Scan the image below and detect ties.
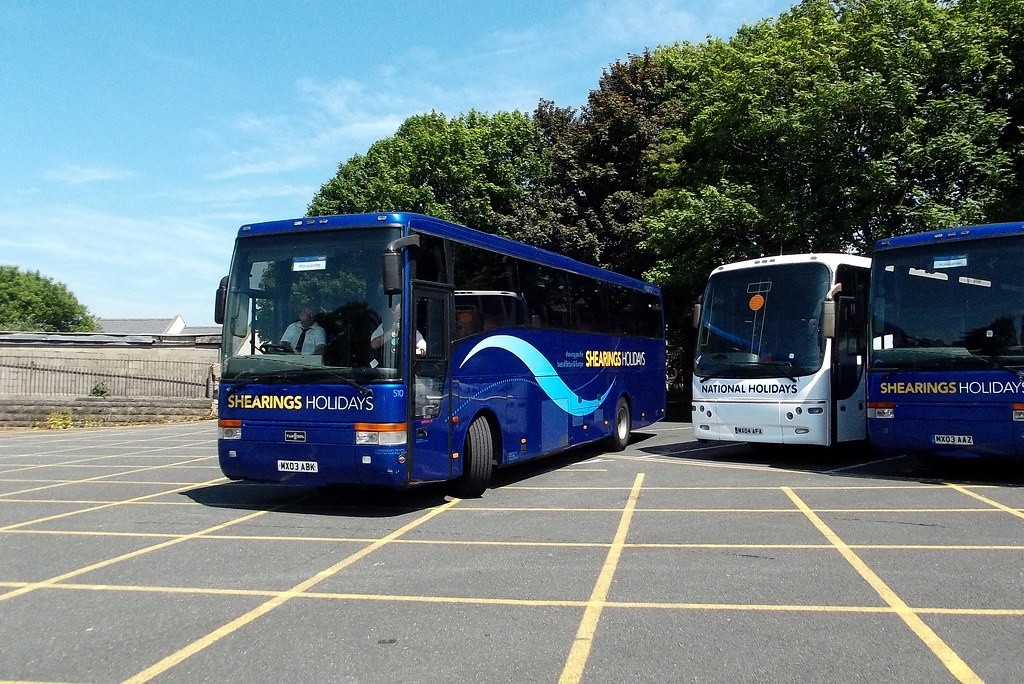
[295,328,311,353]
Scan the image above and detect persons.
[277,304,326,358]
[369,302,427,366]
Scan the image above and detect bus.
[213,211,667,505]
[863,221,1023,484]
[692,252,993,451]
